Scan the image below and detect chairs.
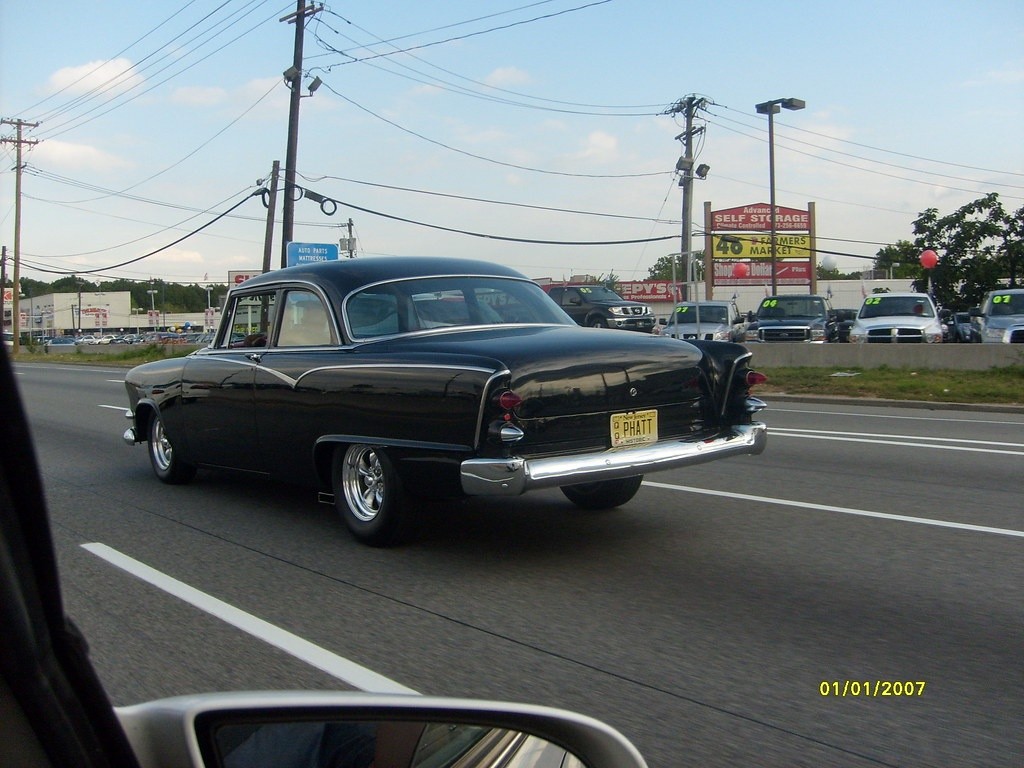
[810,305,822,315]
[770,308,786,316]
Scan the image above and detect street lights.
[205,287,212,332]
[147,290,158,333]
[755,98,805,296]
[132,308,143,335]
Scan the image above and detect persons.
[282,307,327,345]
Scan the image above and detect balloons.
[167,322,190,333]
[918,250,939,268]
[734,263,747,278]
[174,323,179,329]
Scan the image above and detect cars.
[755,294,840,342]
[969,289,1024,343]
[849,292,943,343]
[661,299,741,342]
[123,258,767,544]
[739,313,756,326]
[412,296,504,326]
[34,332,246,345]
[941,311,972,343]
[835,307,859,341]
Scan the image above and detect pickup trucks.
[491,286,660,335]
